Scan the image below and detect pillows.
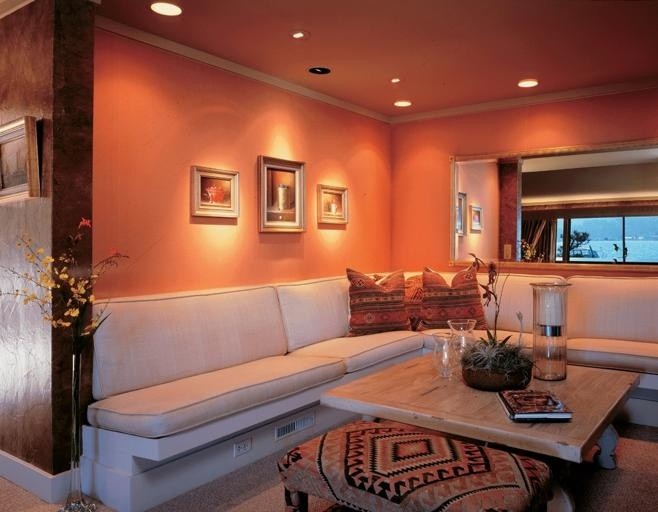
[347,267,488,337]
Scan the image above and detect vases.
[463,367,532,392]
[58,354,96,512]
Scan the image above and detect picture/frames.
[0,116,41,202]
[257,155,306,232]
[317,184,348,225]
[457,192,468,237]
[191,166,239,218]
[469,205,483,233]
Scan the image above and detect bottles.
[530,282,571,381]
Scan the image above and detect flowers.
[0,216,130,355]
[463,253,543,382]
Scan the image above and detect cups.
[432,318,475,379]
[277,187,290,210]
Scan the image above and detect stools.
[277,420,554,512]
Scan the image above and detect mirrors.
[448,138,658,273]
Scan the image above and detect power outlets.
[234,438,252,458]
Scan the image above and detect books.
[498,389,574,421]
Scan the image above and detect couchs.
[80,272,422,512]
[423,273,658,428]
